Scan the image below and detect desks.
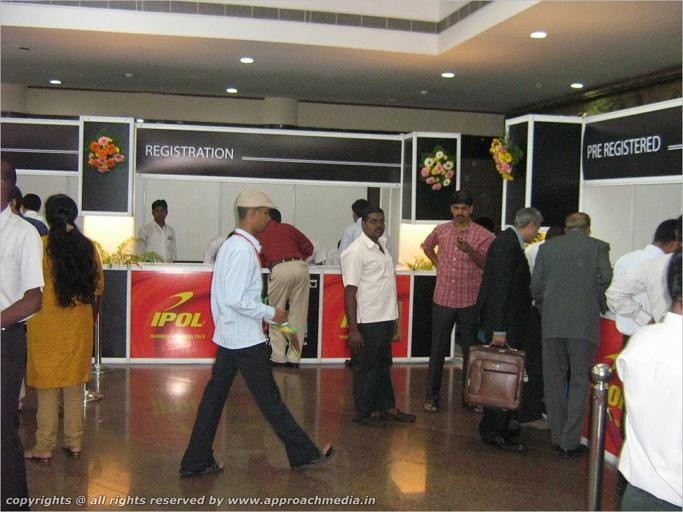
[91,262,455,362]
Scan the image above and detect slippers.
[23,447,52,467]
[61,439,81,461]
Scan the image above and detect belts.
[268,256,306,271]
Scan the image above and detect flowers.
[418,146,456,192]
[489,139,524,183]
[88,130,123,174]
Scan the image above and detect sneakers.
[356,414,389,428]
[379,406,417,422]
[518,416,550,431]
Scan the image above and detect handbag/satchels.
[464,341,529,412]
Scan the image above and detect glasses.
[364,218,386,226]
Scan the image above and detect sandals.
[179,460,225,476]
[290,444,336,472]
[423,394,439,413]
[463,403,483,414]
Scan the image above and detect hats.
[236,188,278,209]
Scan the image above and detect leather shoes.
[562,445,581,465]
[268,359,288,367]
[344,359,352,368]
[285,362,299,368]
[481,428,526,452]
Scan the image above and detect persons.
[475,208,549,450]
[8,185,48,237]
[21,193,51,232]
[422,189,496,414]
[22,193,105,467]
[604,219,683,510]
[133,188,416,477]
[531,212,614,459]
[525,226,565,276]
[605,214,682,326]
[1,155,46,511]
[614,246,683,511]
